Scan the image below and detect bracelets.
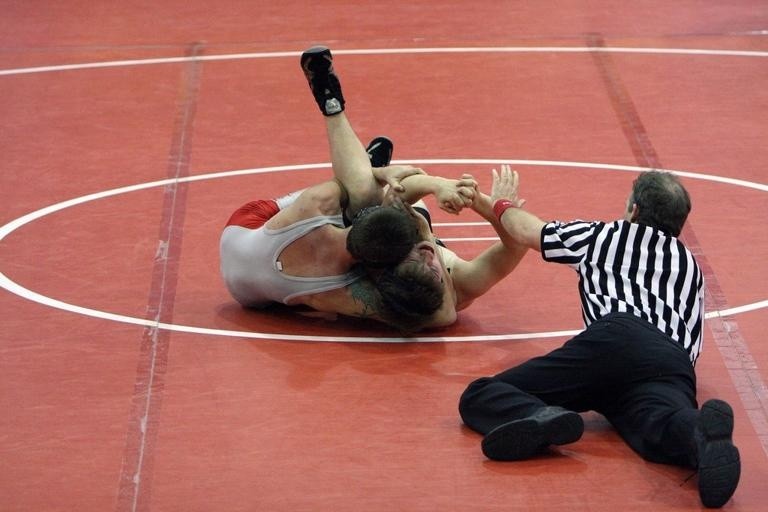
[492,198,521,225]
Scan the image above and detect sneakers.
[301,47,346,117]
[366,137,392,169]
[693,400,740,509]
[480,406,583,461]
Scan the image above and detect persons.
[296,43,526,325]
[457,163,742,511]
[217,135,479,329]
